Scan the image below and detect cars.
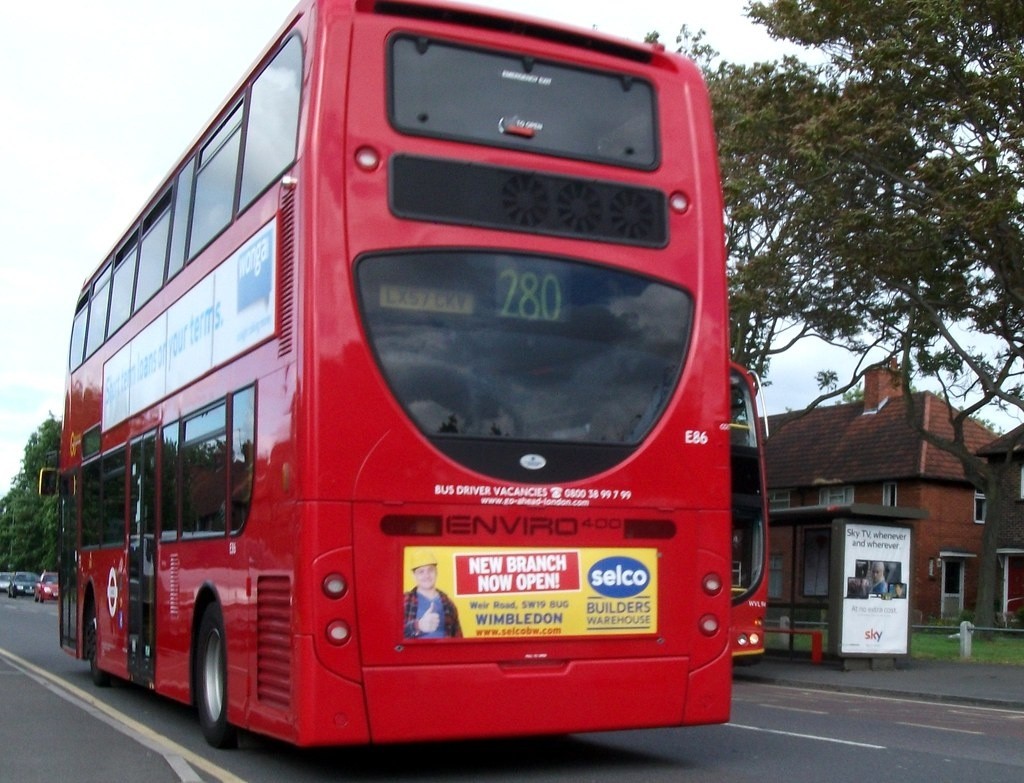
[0,572,12,591]
[35,571,60,603]
[8,571,42,598]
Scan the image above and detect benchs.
[765,626,824,665]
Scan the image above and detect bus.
[724,360,770,667]
[38,0,733,749]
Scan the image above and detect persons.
[849,578,868,598]
[894,582,906,598]
[866,562,888,595]
[402,548,464,639]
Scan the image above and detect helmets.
[409,550,437,570]
